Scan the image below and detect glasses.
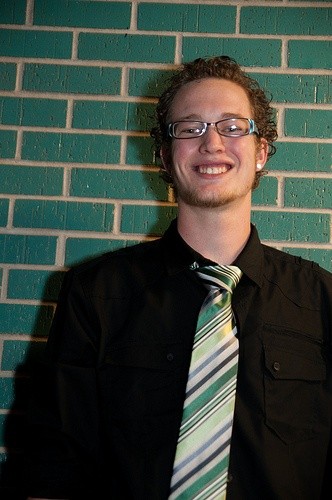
[164,117,260,139]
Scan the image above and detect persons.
[32,55,332,500]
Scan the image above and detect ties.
[165,262,242,499]
